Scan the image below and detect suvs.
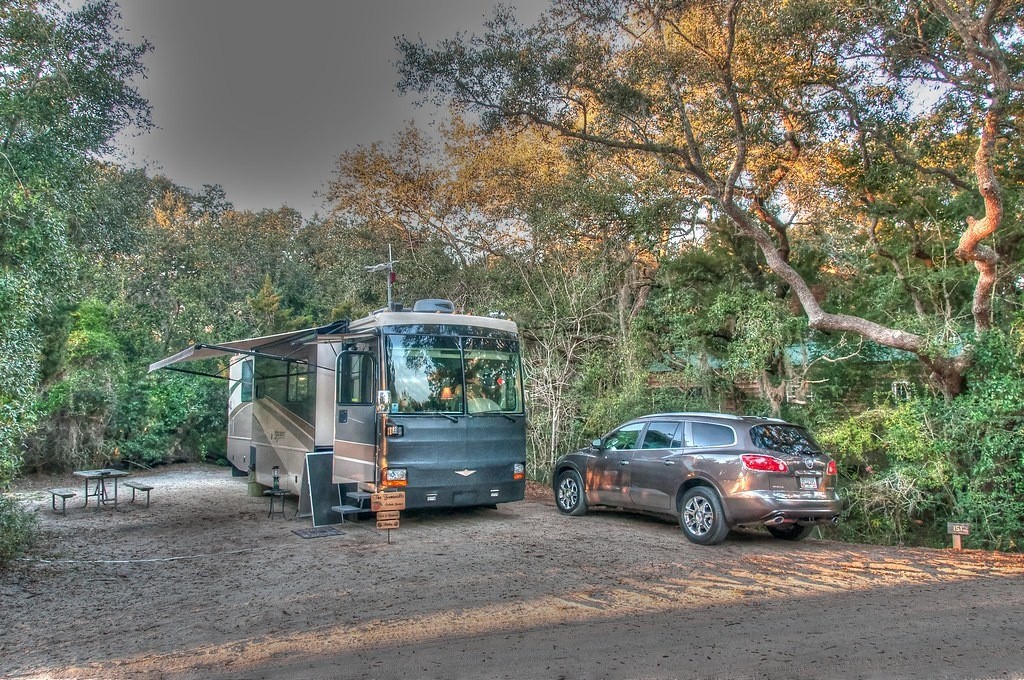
[551,412,843,547]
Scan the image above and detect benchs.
[123,481,154,508]
[48,489,76,517]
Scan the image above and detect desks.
[73,469,132,513]
[263,488,291,519]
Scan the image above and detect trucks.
[146,297,529,515]
